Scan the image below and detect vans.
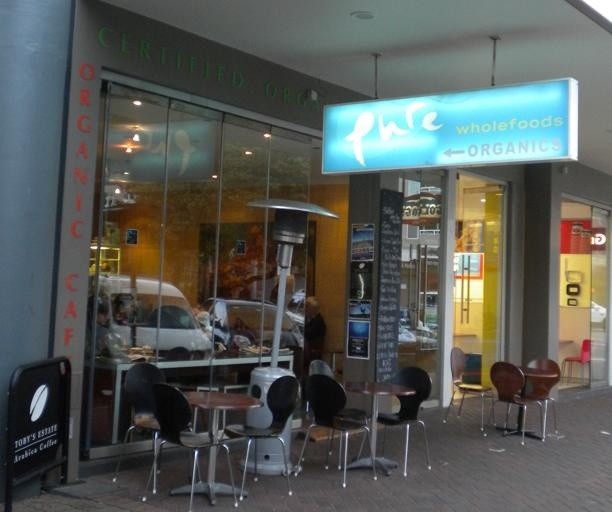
[83,274,306,395]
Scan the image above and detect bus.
[414,291,439,322]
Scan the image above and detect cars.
[396,307,440,357]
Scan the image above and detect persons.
[86,295,112,358]
[303,295,327,369]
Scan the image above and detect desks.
[169,391,264,504]
[85,349,295,445]
[346,380,417,470]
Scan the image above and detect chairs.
[440,347,497,430]
[111,363,202,485]
[294,374,377,488]
[561,338,592,385]
[308,358,371,469]
[356,365,432,476]
[223,375,302,500]
[142,384,239,510]
[485,360,562,445]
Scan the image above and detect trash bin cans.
[239,367,302,476]
